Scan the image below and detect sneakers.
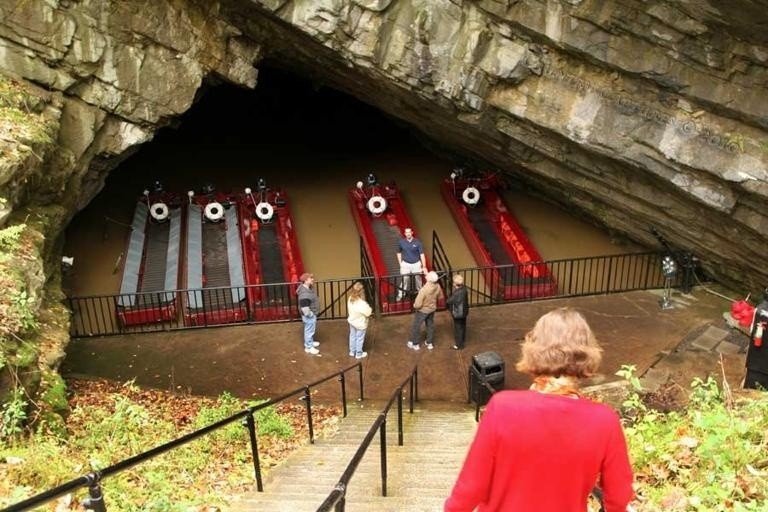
[304,341,320,354]
[349,351,367,360]
[424,340,434,350]
[407,341,420,351]
[395,296,402,302]
[453,345,458,350]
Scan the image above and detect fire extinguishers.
[754,321,767,347]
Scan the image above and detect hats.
[299,273,314,281]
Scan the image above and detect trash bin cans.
[468,351,505,406]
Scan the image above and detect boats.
[112,180,183,328]
[236,180,307,324]
[439,168,560,300]
[183,184,249,329]
[347,173,449,316]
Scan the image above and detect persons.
[297,272,322,356]
[395,227,428,302]
[412,271,444,346]
[446,274,469,350]
[347,282,373,360]
[443,306,635,512]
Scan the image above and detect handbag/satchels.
[452,304,464,319]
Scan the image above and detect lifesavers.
[203,202,225,219]
[463,188,478,203]
[367,196,387,213]
[151,203,168,220]
[256,203,273,221]
